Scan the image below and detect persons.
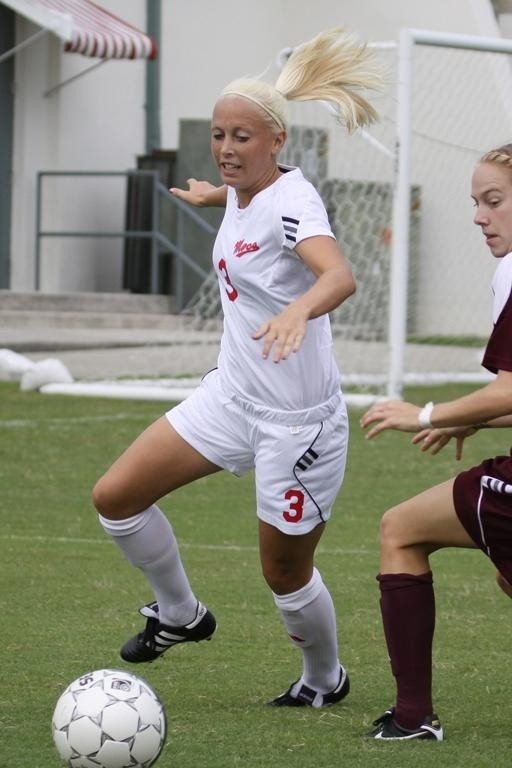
[85,24,390,711]
[356,141,512,742]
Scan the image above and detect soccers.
[51,669,167,768]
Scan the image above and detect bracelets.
[420,400,436,430]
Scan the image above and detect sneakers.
[263,665,352,713]
[355,706,446,745]
[118,600,217,663]
